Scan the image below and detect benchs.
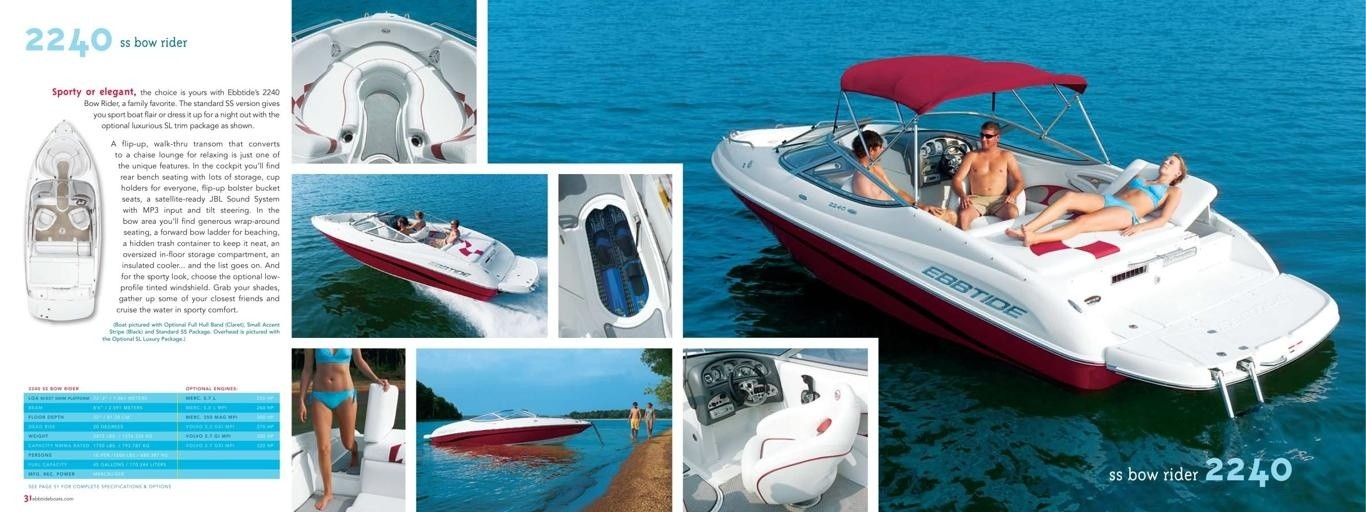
[402,226,429,244]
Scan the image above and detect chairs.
[840,156,1219,280]
[741,382,861,510]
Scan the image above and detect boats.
[423,408,593,451]
[708,53,1333,421]
[310,211,540,303]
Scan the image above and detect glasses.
[981,133,996,139]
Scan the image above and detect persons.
[1006,153,1186,248]
[853,130,958,226]
[628,402,642,440]
[953,122,1025,231]
[397,216,409,236]
[297,348,390,511]
[428,220,459,249]
[644,403,656,434]
[405,212,426,232]
